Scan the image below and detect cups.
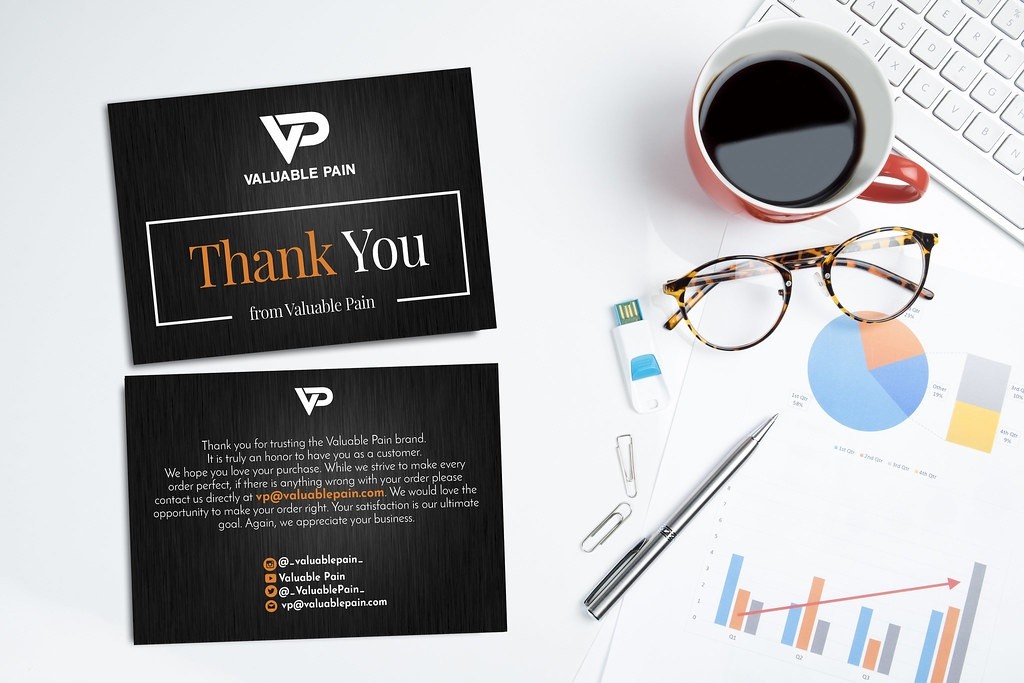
[685,18,927,225]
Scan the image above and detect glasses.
[661,226,938,351]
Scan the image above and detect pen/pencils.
[584,413,779,621]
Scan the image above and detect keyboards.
[744,0,1024,246]
